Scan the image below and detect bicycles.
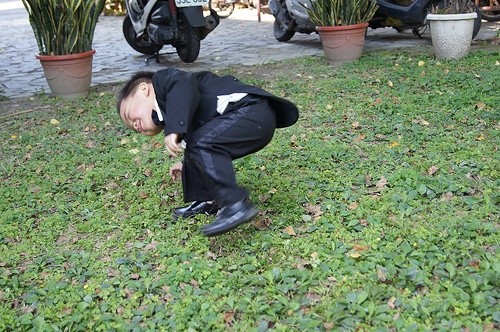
[208,0,266,22]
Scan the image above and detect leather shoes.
[200,199,259,235]
[173,200,218,221]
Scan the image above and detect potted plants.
[297,0,380,66]
[21,0,106,98]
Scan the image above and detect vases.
[426,12,478,61]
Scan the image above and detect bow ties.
[152,110,165,125]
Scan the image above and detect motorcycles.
[121,0,219,64]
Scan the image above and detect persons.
[117,67,299,237]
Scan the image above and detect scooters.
[267,0,500,42]
